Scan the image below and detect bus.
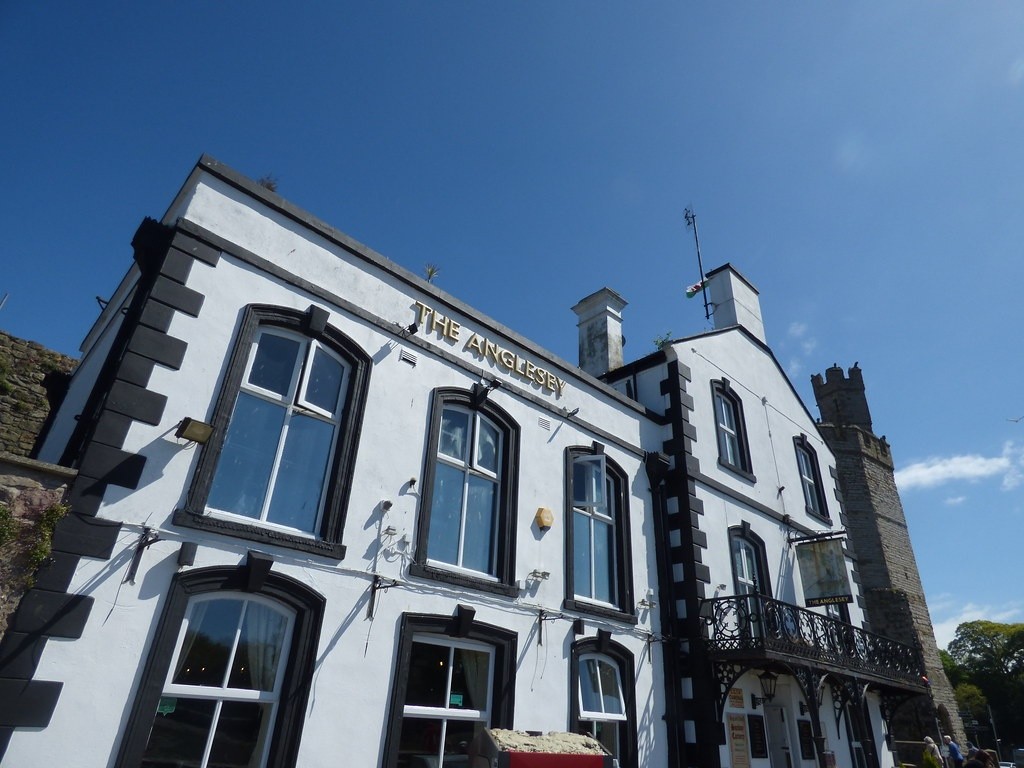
[1012,748,1024,768]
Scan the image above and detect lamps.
[751,671,779,709]
[573,619,584,636]
[177,541,198,566]
[485,379,502,393]
[406,323,418,335]
[799,685,826,716]
[174,417,216,445]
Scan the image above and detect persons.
[924,736,944,768]
[944,735,996,768]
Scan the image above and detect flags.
[686,275,709,299]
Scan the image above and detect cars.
[999,761,1017,768]
